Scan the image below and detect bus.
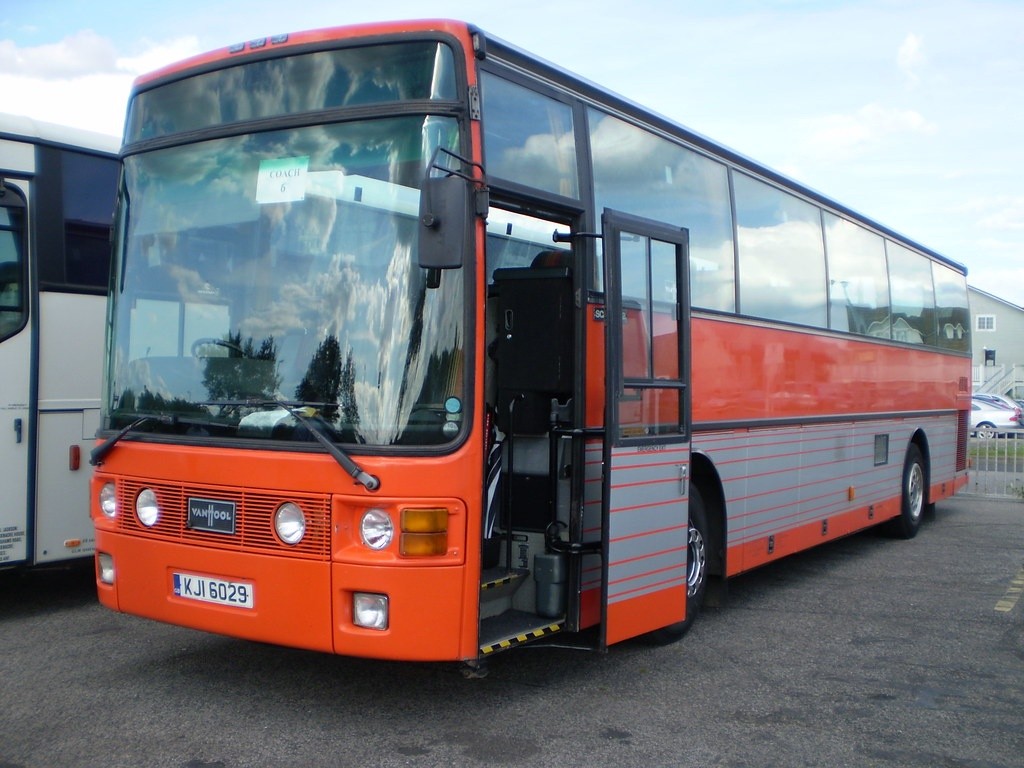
[0,113,572,576]
[89,16,974,666]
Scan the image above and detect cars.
[970,393,1024,439]
